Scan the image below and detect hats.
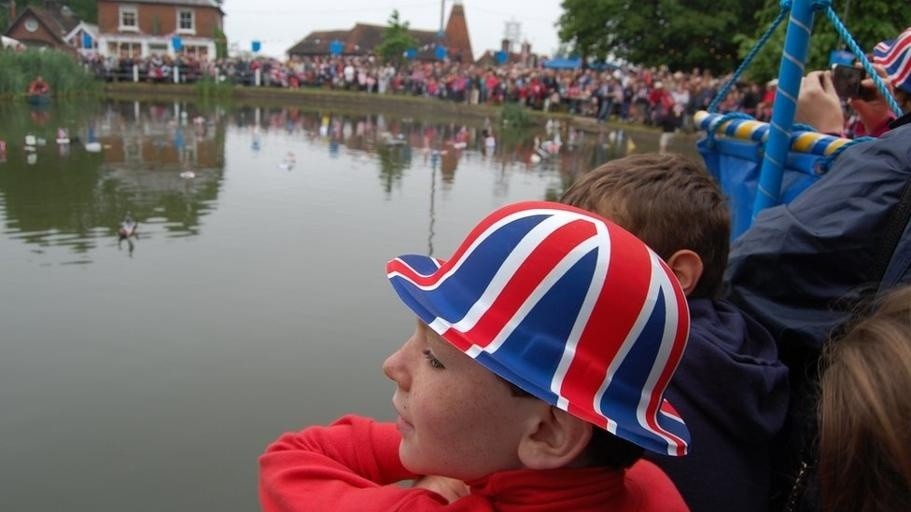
[871,27,911,93]
[383,198,697,456]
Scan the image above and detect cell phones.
[831,63,872,101]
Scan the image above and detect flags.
[252,42,260,52]
[331,40,342,56]
[873,29,911,87]
[83,34,92,48]
[172,36,181,53]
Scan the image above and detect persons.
[814,283,910,511]
[554,152,791,511]
[723,108,910,511]
[1,25,911,140]
[255,197,694,512]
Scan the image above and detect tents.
[402,44,447,61]
[543,58,620,73]
